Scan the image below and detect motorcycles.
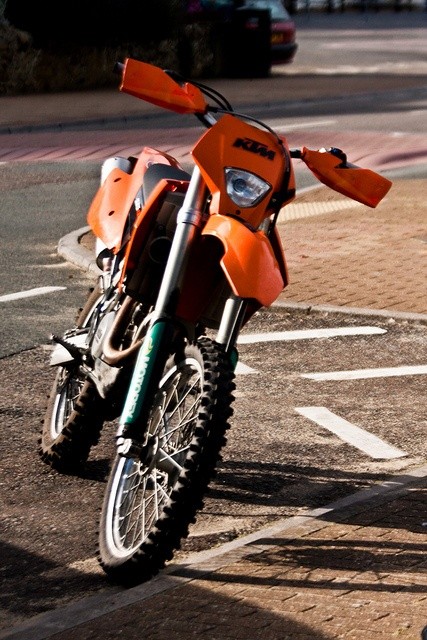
[38,58,391,584]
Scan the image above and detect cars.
[241,0,297,63]
